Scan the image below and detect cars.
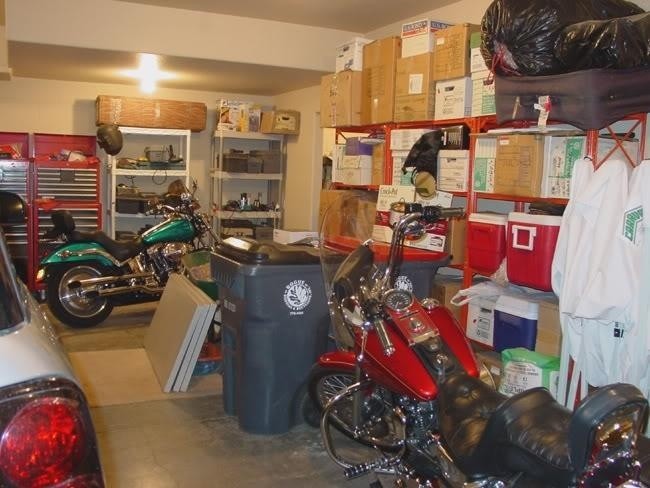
[0,190,104,486]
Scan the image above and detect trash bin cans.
[211,238,344,434]
[323,236,453,424]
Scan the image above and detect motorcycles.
[34,177,222,328]
[309,187,649,488]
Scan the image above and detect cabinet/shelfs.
[210,130,284,246]
[105,125,190,241]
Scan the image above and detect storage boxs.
[216,98,262,131]
[95,94,207,133]
[261,110,300,134]
[319,19,646,398]
